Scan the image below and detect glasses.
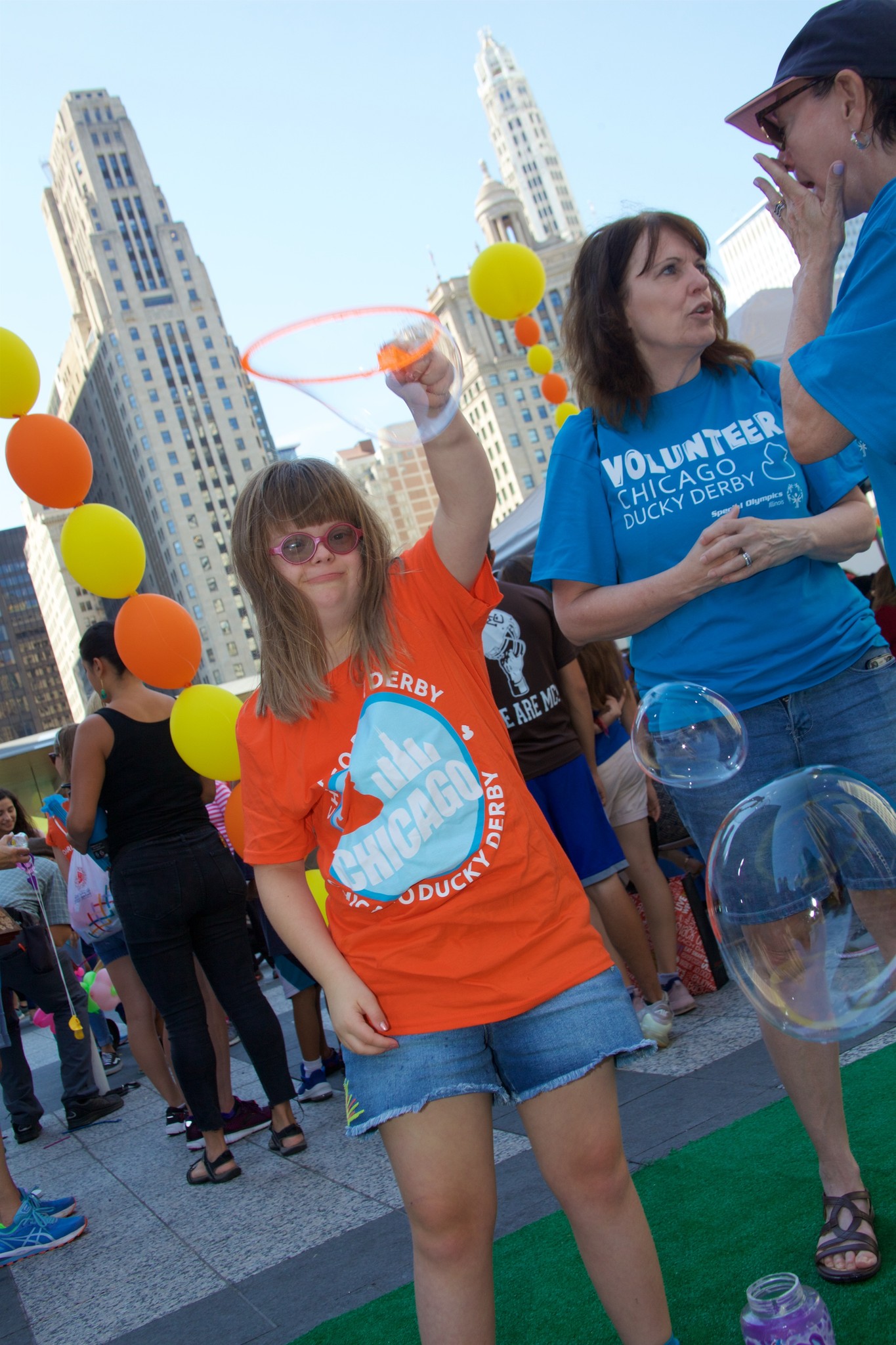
[48,752,60,765]
[269,523,363,565]
[755,76,827,151]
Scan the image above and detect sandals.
[268,1121,307,1156]
[186,1149,241,1185]
[813,1187,881,1284]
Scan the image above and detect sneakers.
[4,1113,42,1144]
[65,1094,124,1131]
[17,1185,76,1218]
[0,1200,88,1267]
[101,1052,123,1075]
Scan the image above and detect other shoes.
[666,980,696,1016]
[300,1047,341,1079]
[185,1115,206,1150]
[165,1104,190,1135]
[635,990,675,1047]
[297,1068,333,1103]
[221,1095,272,1145]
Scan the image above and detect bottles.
[739,1270,836,1345]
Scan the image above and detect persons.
[529,210,896,1284]
[873,564,896,658]
[0,616,344,1269]
[476,530,695,1048]
[723,0,896,583]
[232,329,678,1345]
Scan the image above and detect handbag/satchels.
[608,870,729,997]
[67,847,122,945]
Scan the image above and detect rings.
[741,550,753,567]
[774,199,787,217]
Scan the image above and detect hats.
[724,0,896,146]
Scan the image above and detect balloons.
[526,343,553,375]
[553,401,582,430]
[113,593,202,690]
[5,414,94,509]
[225,781,255,867]
[0,325,41,419]
[515,317,540,345]
[170,684,243,782]
[60,502,147,599]
[542,374,568,403]
[469,244,549,322]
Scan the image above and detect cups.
[6,832,29,848]
